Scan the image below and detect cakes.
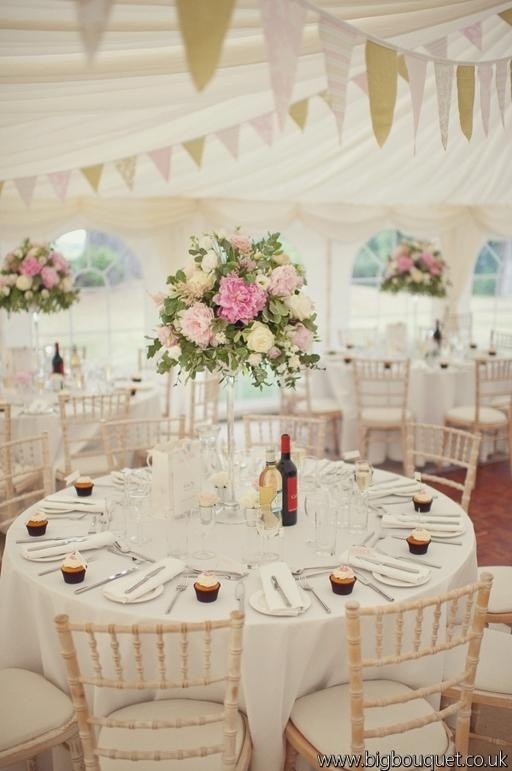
[60,549,87,584]
[412,492,433,511]
[193,571,221,602]
[75,475,94,496]
[329,565,357,595]
[406,526,431,555]
[25,511,48,537]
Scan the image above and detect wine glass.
[125,436,374,561]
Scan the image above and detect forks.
[165,575,189,616]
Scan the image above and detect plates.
[21,508,165,603]
[248,493,465,617]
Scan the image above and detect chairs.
[0,667,85,771]
[441,358,511,473]
[247,412,327,455]
[477,565,512,628]
[402,422,482,517]
[53,611,251,771]
[284,572,494,771]
[0,433,51,532]
[277,369,341,458]
[51,390,130,481]
[165,379,219,435]
[351,356,415,467]
[442,629,512,770]
[103,414,186,472]
[0,402,45,520]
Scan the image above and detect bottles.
[259,433,298,538]
[433,320,441,351]
[69,345,82,388]
[52,343,64,390]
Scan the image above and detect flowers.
[0,238,82,315]
[380,238,452,300]
[142,225,319,386]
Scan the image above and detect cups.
[105,457,368,574]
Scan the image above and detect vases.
[214,380,252,526]
